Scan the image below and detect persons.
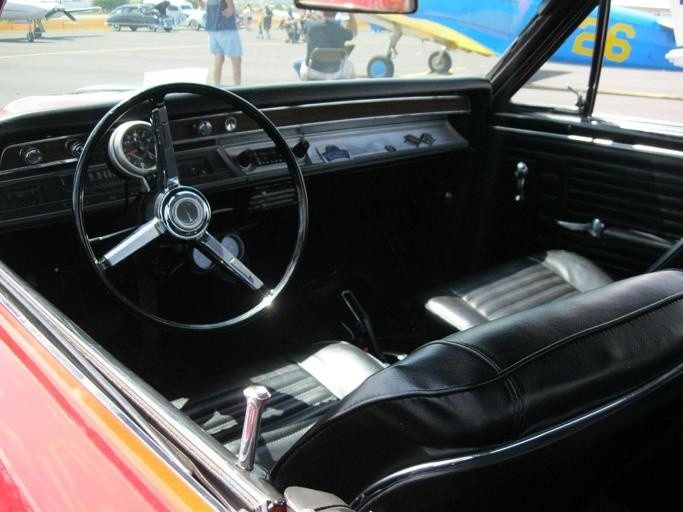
[195,0,244,89]
[234,4,320,45]
[293,9,358,81]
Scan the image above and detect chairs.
[164,268,683,508]
[398,251,616,337]
[299,48,346,81]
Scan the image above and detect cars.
[105,1,207,32]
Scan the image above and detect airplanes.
[336,0,683,79]
[0,0,102,42]
